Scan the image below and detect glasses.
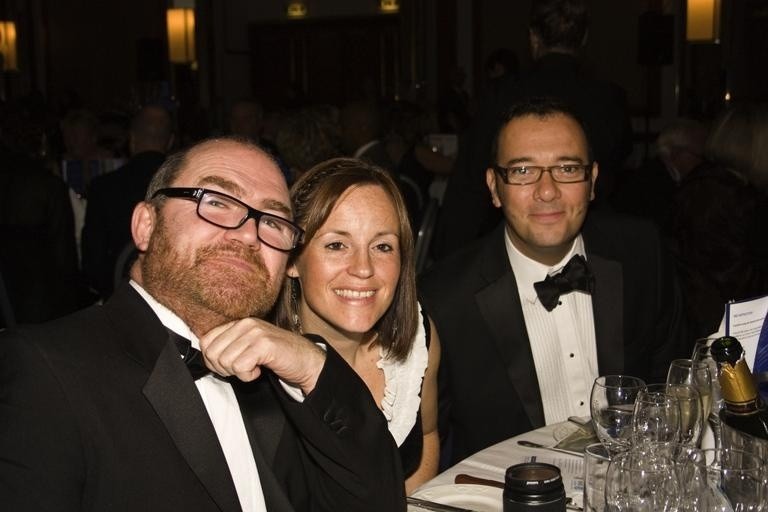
[149,186,303,253]
[493,164,589,186]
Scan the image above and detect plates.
[407,483,505,512]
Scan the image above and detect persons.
[2,128,405,511]
[1,3,766,382]
[413,86,670,473]
[273,156,446,501]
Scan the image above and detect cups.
[582,335,767,511]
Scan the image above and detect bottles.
[709,336,767,442]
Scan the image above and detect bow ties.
[533,254,590,313]
[184,349,211,380]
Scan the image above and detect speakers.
[636,11,673,65]
[136,38,166,81]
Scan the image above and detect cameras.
[503,462,566,512]
